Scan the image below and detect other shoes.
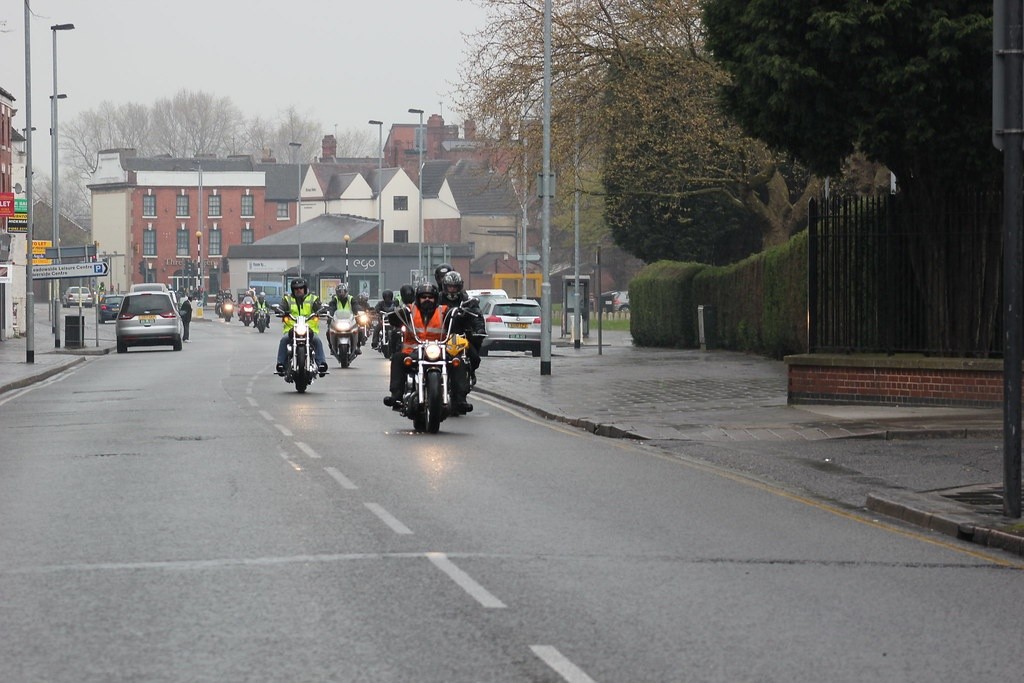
[355,349,362,354]
[455,395,473,412]
[266,323,270,328]
[371,342,378,348]
[253,324,256,328]
[276,363,285,376]
[330,349,334,355]
[384,392,404,406]
[319,362,328,377]
[184,340,191,342]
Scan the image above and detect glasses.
[420,294,435,299]
[447,285,458,288]
[294,287,303,289]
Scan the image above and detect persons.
[175,286,209,306]
[383,283,473,413]
[276,278,328,378]
[238,289,271,328]
[435,263,486,370]
[214,290,234,317]
[327,284,365,355]
[180,295,193,341]
[99,282,106,291]
[356,291,373,340]
[371,289,395,347]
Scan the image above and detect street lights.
[49,92,69,333]
[290,141,303,278]
[188,168,205,301]
[51,23,77,348]
[368,119,384,299]
[407,107,424,287]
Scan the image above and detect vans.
[130,283,177,308]
[465,288,508,319]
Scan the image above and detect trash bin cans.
[64,315,85,349]
[697,304,719,349]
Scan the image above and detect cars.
[589,290,630,314]
[479,297,542,357]
[98,294,130,325]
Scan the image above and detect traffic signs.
[31,261,110,280]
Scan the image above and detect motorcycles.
[271,303,330,392]
[320,310,394,368]
[387,297,490,435]
[213,295,271,334]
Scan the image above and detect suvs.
[62,286,94,309]
[113,290,187,353]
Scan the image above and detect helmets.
[400,285,414,303]
[335,283,348,299]
[358,292,369,301]
[382,289,393,300]
[435,263,454,284]
[291,278,307,294]
[416,282,439,310]
[442,271,463,301]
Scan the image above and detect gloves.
[454,308,465,320]
[319,310,328,318]
[396,308,410,324]
[476,327,486,339]
